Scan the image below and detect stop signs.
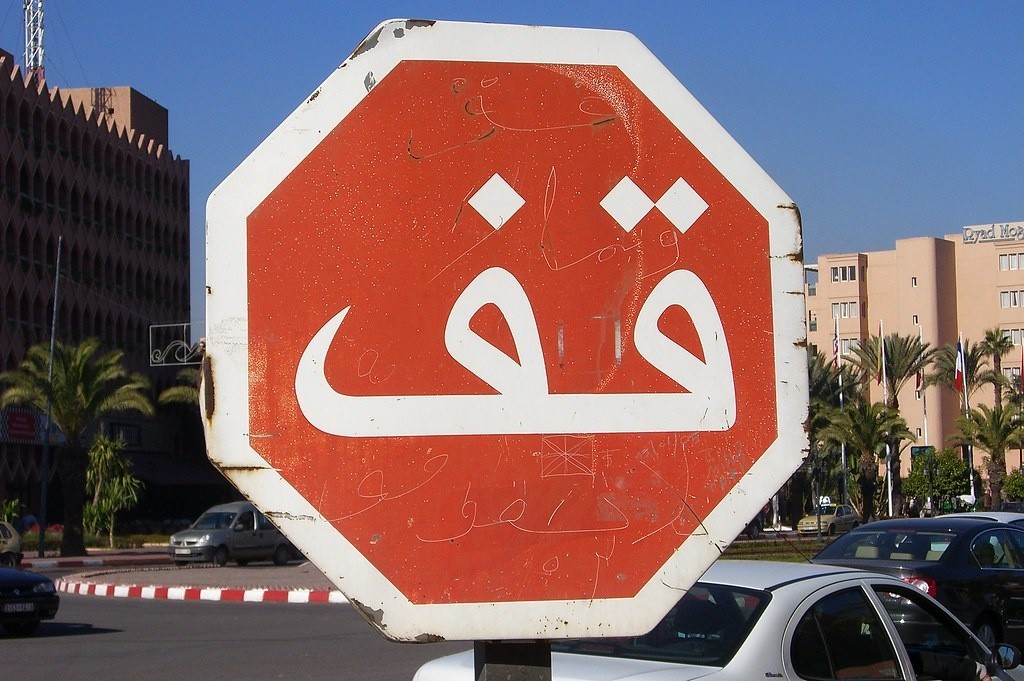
[198,16,811,643]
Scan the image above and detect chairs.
[974,542,995,568]
[855,545,879,559]
[669,614,731,653]
[890,553,913,560]
[925,550,944,561]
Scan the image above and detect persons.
[982,490,991,509]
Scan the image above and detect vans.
[167,500,294,568]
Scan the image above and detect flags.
[877,324,887,386]
[914,341,921,401]
[954,337,966,393]
[832,337,838,367]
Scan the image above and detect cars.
[742,501,774,540]
[0,567,60,628]
[797,496,860,537]
[0,522,24,570]
[415,511,1024,681]
